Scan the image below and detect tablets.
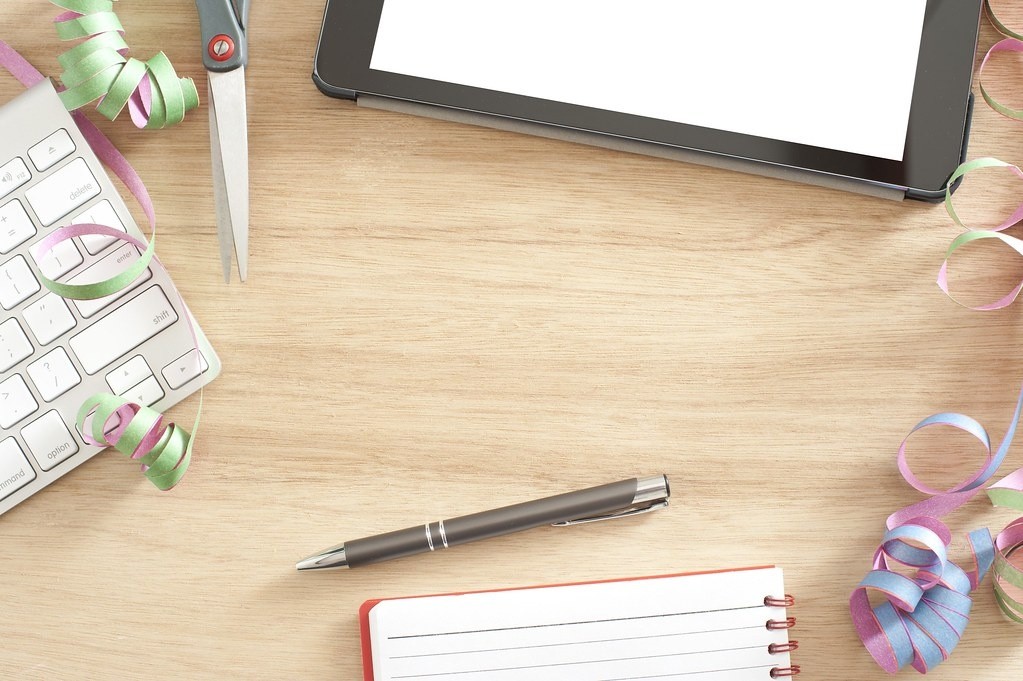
[311,0,982,204]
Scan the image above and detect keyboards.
[1,76,222,524]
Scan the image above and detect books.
[356,566,802,679]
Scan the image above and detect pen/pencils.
[294,473,672,574]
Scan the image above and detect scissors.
[190,0,254,285]
